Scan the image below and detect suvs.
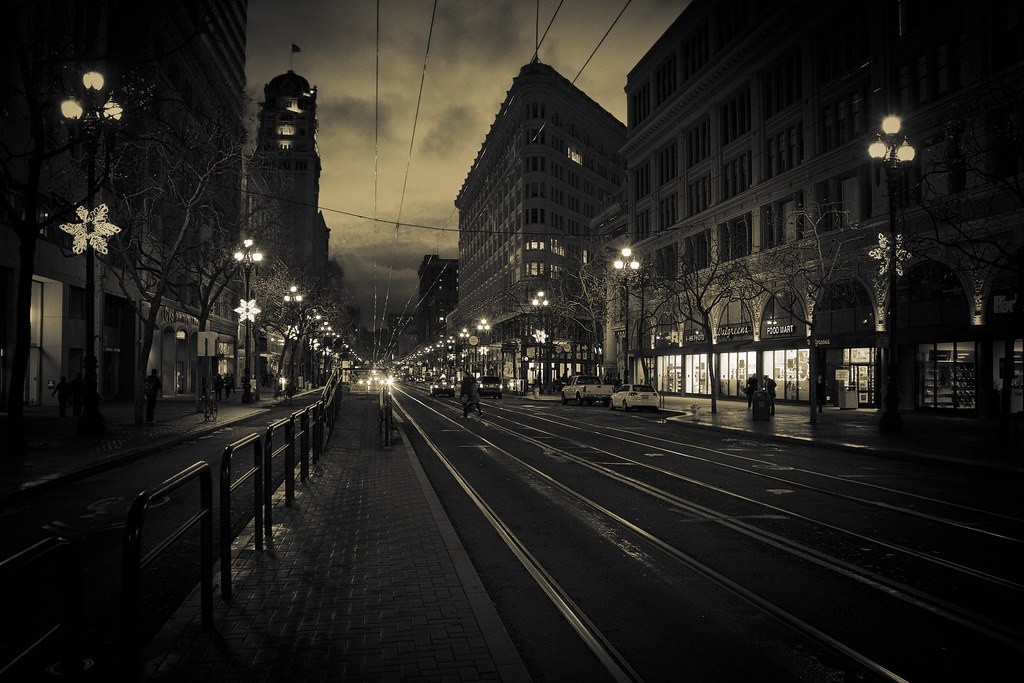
[477,375,505,398]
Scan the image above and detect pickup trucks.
[561,373,615,405]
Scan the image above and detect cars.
[609,383,660,413]
[428,378,456,396]
[380,370,426,383]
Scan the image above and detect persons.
[52,373,84,416]
[262,370,274,388]
[460,375,483,418]
[144,368,162,422]
[816,372,826,414]
[300,364,304,376]
[537,372,572,392]
[214,373,238,402]
[746,374,777,418]
[274,368,297,397]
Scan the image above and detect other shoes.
[478,414,484,418]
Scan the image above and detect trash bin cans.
[753,390,771,421]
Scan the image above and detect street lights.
[234,238,264,404]
[460,328,471,377]
[615,245,640,383]
[282,284,303,396]
[478,318,492,377]
[532,290,546,392]
[866,111,917,440]
[59,70,123,440]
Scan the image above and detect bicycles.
[201,391,219,422]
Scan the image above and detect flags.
[291,42,300,53]
